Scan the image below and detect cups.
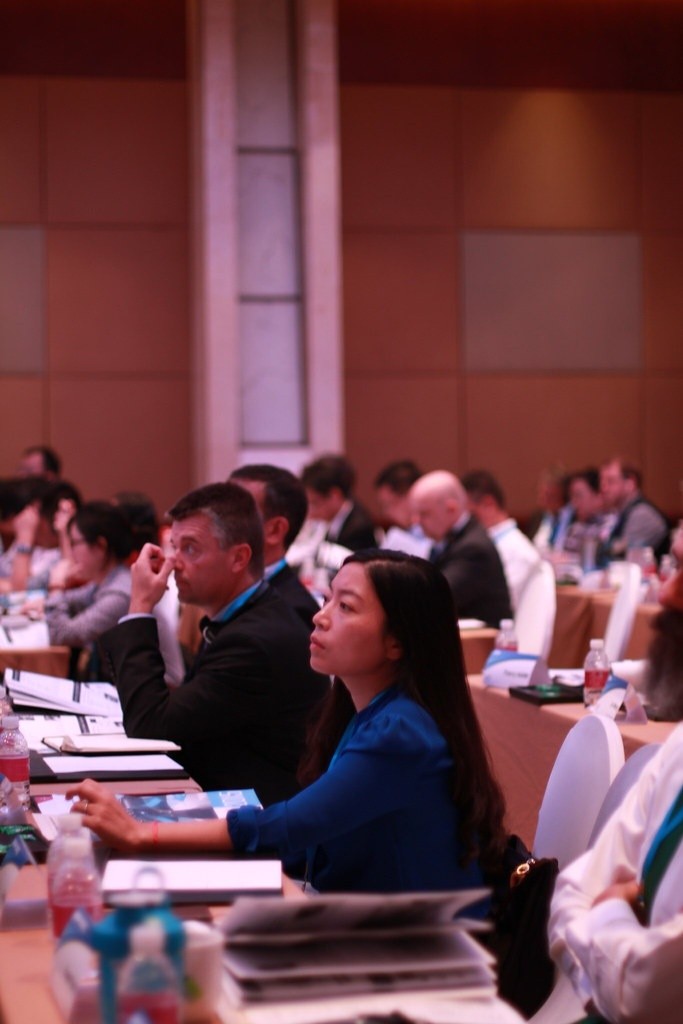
[180,921,225,1016]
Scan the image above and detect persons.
[0,444,683,1024]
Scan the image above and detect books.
[98,856,286,900]
[204,888,523,1023]
[41,735,182,754]
[28,781,270,845]
[1,667,126,726]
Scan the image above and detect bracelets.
[48,585,64,590]
[152,820,159,844]
[15,544,33,553]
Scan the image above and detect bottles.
[116,921,178,1024]
[638,547,656,585]
[47,813,96,889]
[51,839,103,940]
[583,639,608,708]
[0,716,31,813]
[0,685,12,732]
[659,555,672,584]
[494,618,517,652]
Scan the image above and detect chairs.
[512,561,558,662]
[532,712,625,872]
[590,744,663,850]
[603,564,643,664]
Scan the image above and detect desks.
[0,646,307,1024]
[458,571,678,853]
